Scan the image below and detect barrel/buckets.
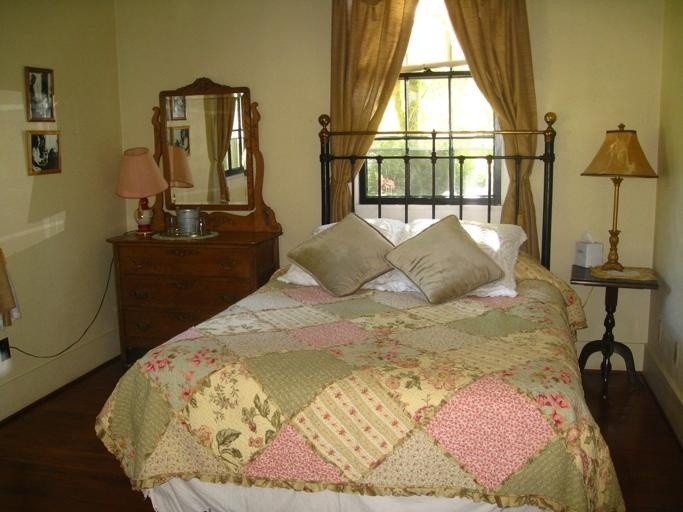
[175,206,201,234]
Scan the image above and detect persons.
[172,97,189,154]
[29,73,58,171]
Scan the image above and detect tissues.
[576,232,604,268]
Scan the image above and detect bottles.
[199,217,206,236]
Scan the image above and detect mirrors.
[146,78,282,234]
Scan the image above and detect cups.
[168,224,180,237]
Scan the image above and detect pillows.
[385,208,505,305]
[396,216,528,300]
[288,210,395,301]
[277,218,403,300]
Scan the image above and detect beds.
[92,112,630,511]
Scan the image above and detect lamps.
[582,127,661,272]
[113,145,168,234]
[167,144,194,204]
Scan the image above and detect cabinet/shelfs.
[111,232,278,366]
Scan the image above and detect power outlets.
[0,338,11,362]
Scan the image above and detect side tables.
[570,263,659,437]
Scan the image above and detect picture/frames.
[169,96,186,121]
[26,130,63,178]
[25,65,56,123]
[170,126,190,159]
